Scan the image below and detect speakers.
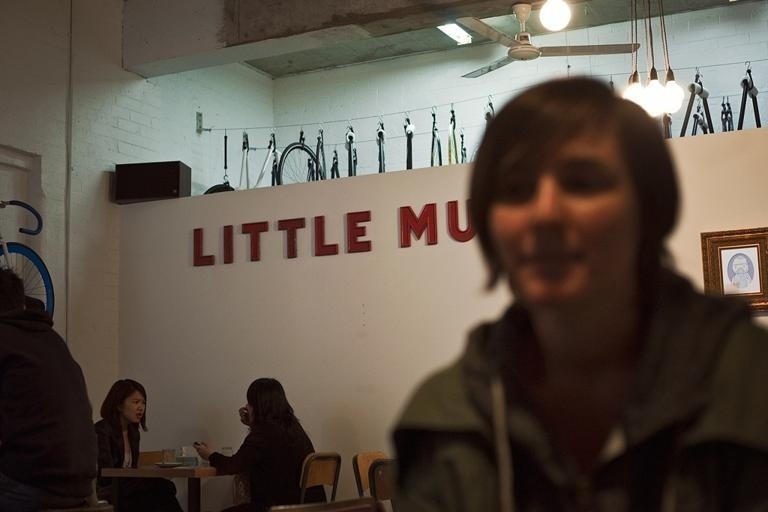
[116,160,191,203]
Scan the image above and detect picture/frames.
[700,227,768,311]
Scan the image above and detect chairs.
[352,451,387,510]
[268,495,386,511]
[139,451,174,467]
[299,451,342,504]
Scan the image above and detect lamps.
[622,0,684,119]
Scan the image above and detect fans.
[456,1,640,78]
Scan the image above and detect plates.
[155,462,182,467]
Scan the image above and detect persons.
[385,72,767,508]
[1,266,98,511]
[192,378,329,512]
[92,377,186,512]
